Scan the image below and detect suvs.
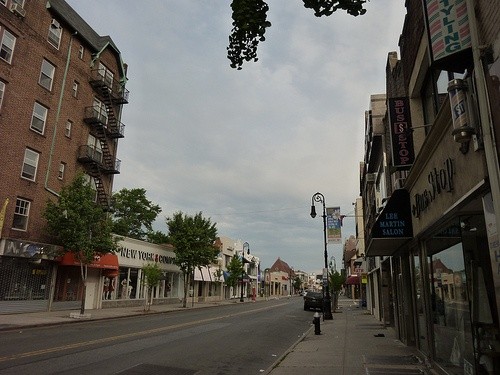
[417,293,444,315]
[304,292,326,312]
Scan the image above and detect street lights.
[240,242,250,302]
[310,192,334,320]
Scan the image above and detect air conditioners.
[9,3,26,18]
[365,173,376,185]
[51,18,60,29]
